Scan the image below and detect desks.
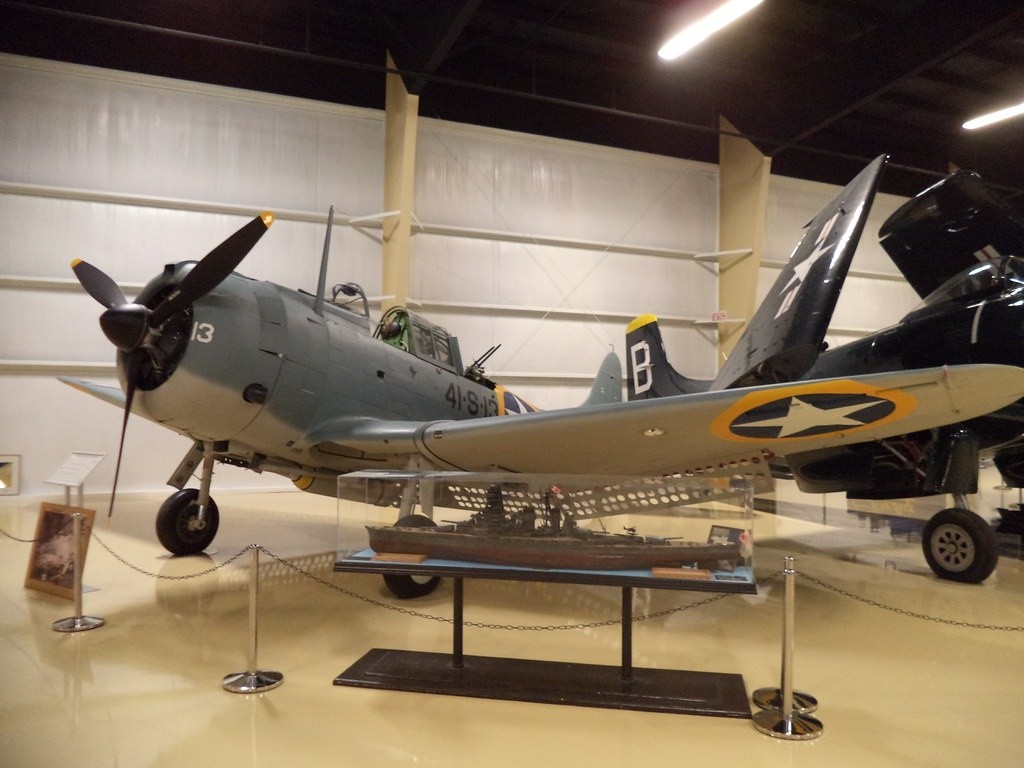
[333,541,756,719]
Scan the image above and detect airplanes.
[71,151,1024,585]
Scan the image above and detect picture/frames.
[24,502,95,600]
[0,454,21,496]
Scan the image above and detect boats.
[365,484,741,571]
